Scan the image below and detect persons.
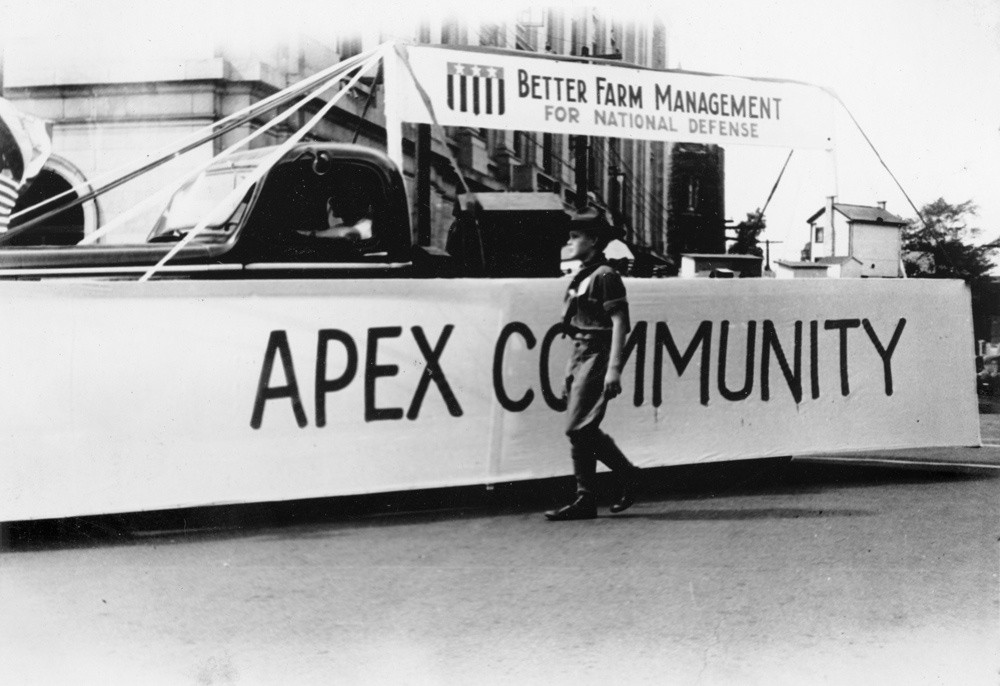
[295,173,375,240]
[542,203,649,521]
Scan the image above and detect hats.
[567,210,610,235]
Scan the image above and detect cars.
[0,144,423,279]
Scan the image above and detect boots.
[596,434,644,512]
[545,444,599,518]
